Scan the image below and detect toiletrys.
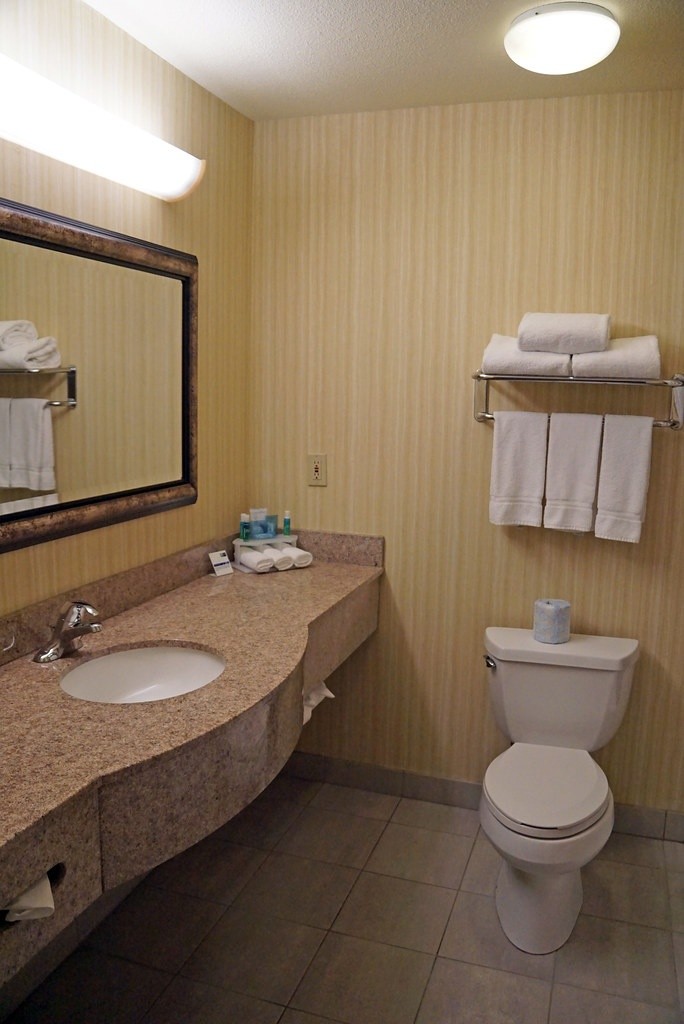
[238,508,292,542]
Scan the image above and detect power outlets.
[307,453,327,486]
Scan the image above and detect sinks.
[57,637,231,705]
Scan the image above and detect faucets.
[35,598,104,664]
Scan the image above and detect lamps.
[504,2,621,75]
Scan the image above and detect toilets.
[473,627,643,956]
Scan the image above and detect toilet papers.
[534,598,572,645]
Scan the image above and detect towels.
[488,409,548,533]
[572,335,663,380]
[3,872,55,924]
[302,681,336,724]
[543,414,606,533]
[274,543,313,568]
[519,311,612,355]
[480,333,572,377]
[594,413,657,543]
[0,320,62,372]
[1,397,56,492]
[233,545,274,574]
[254,544,294,571]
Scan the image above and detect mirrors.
[0,197,200,554]
